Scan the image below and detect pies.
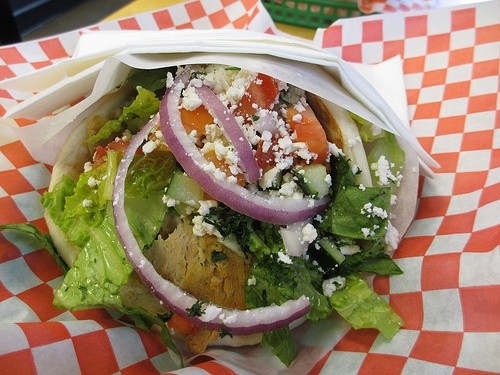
[0,66,420,369]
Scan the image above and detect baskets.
[261,0,383,29]
